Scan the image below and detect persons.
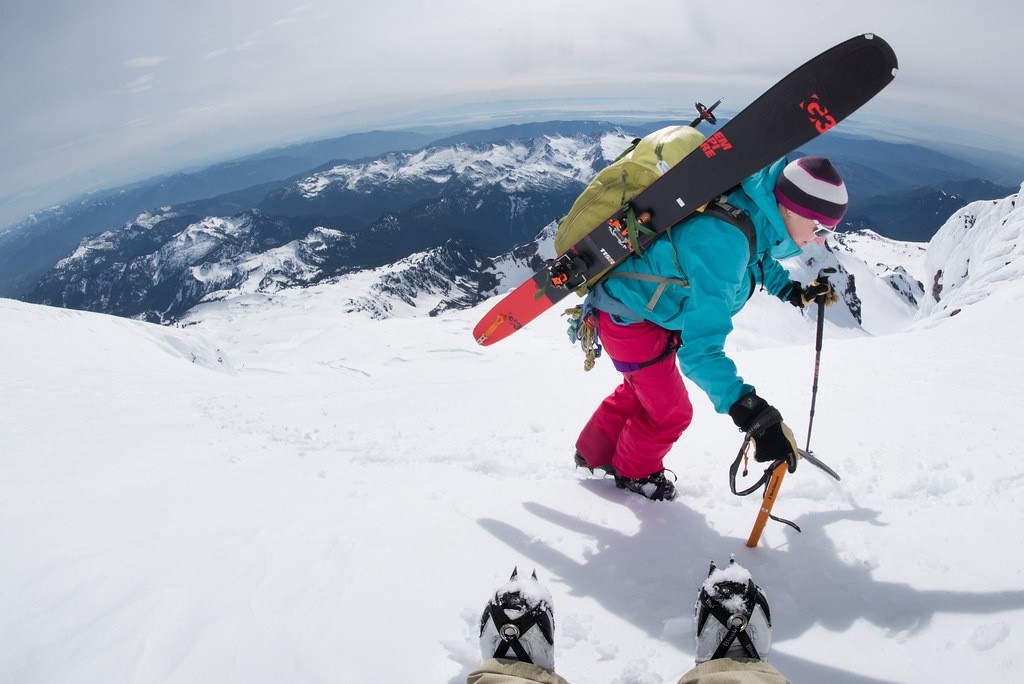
[574,156,850,502]
[467,559,791,684]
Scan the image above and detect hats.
[775,156,847,226]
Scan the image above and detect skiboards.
[472,33,899,346]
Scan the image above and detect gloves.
[790,278,839,308]
[730,393,798,474]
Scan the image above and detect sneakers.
[478,566,558,671]
[574,449,612,474]
[616,474,676,503]
[694,555,772,662]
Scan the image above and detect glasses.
[812,219,833,239]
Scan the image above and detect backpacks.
[553,125,757,299]
